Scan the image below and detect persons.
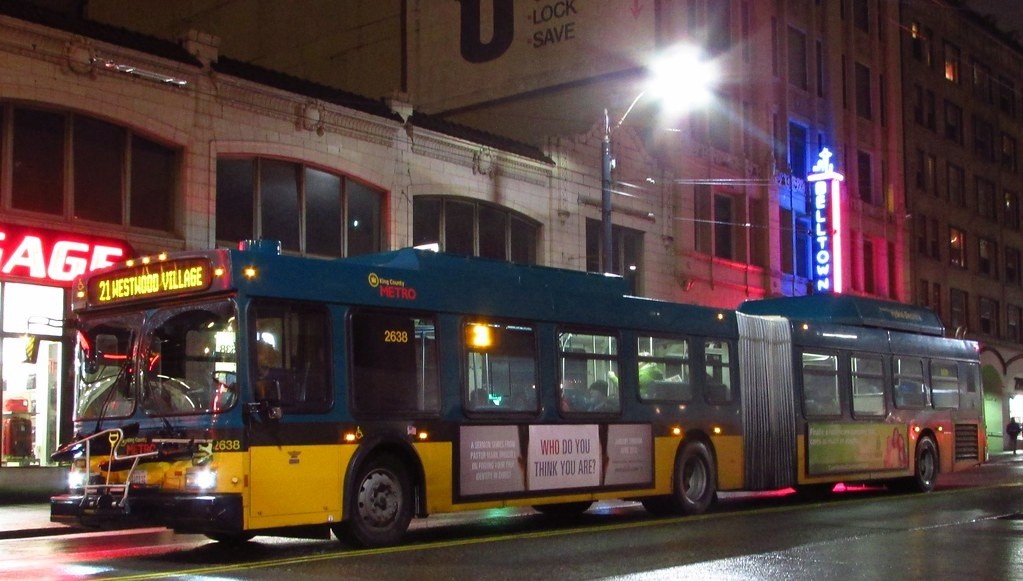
[1005,417,1022,456]
[471,351,734,415]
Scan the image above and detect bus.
[49,239,990,550]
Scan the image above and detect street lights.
[593,38,719,280]
